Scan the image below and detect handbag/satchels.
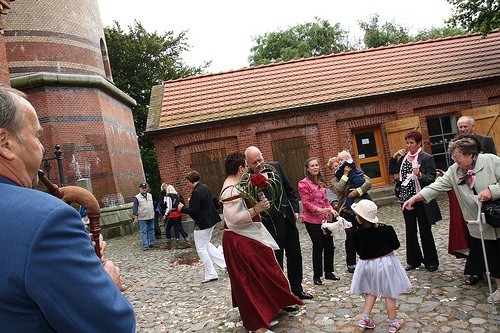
[212,196,221,210]
[481,200,500,229]
[393,177,402,199]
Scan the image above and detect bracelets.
[253,206,258,215]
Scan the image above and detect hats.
[139,183,149,188]
[338,150,353,164]
[351,199,379,223]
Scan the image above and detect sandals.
[388,319,401,333]
[358,318,375,329]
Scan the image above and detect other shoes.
[312,277,322,285]
[405,261,421,271]
[464,274,484,285]
[269,320,279,327]
[283,304,300,312]
[296,291,313,299]
[347,265,355,273]
[487,288,500,303]
[184,241,191,247]
[201,278,218,283]
[249,329,275,333]
[324,273,340,280]
[425,265,437,272]
[165,243,171,249]
[143,245,148,251]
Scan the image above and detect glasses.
[451,154,462,160]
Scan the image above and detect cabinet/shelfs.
[430,131,460,157]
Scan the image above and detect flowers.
[251,171,270,190]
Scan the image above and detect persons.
[298,156,340,285]
[162,185,189,242]
[389,131,442,273]
[0,84,137,333]
[133,182,155,250]
[449,116,497,166]
[335,149,373,208]
[402,138,500,305]
[145,182,162,239]
[327,157,373,273]
[177,171,227,283]
[219,151,304,333]
[245,146,314,306]
[156,182,180,241]
